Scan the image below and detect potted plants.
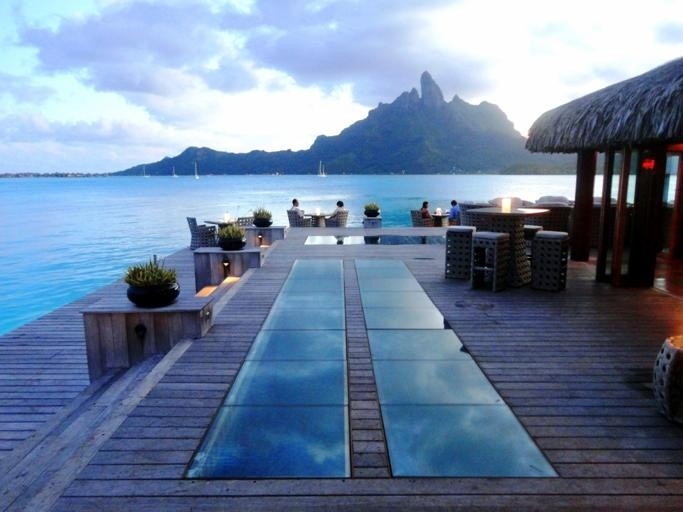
[122,255,180,308]
[363,202,380,218]
[212,206,275,250]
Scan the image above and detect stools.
[443,223,571,297]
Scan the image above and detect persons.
[448,200,460,222]
[327,201,345,223]
[291,199,304,219]
[421,201,434,220]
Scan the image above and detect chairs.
[286,208,350,228]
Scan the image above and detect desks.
[463,207,550,288]
